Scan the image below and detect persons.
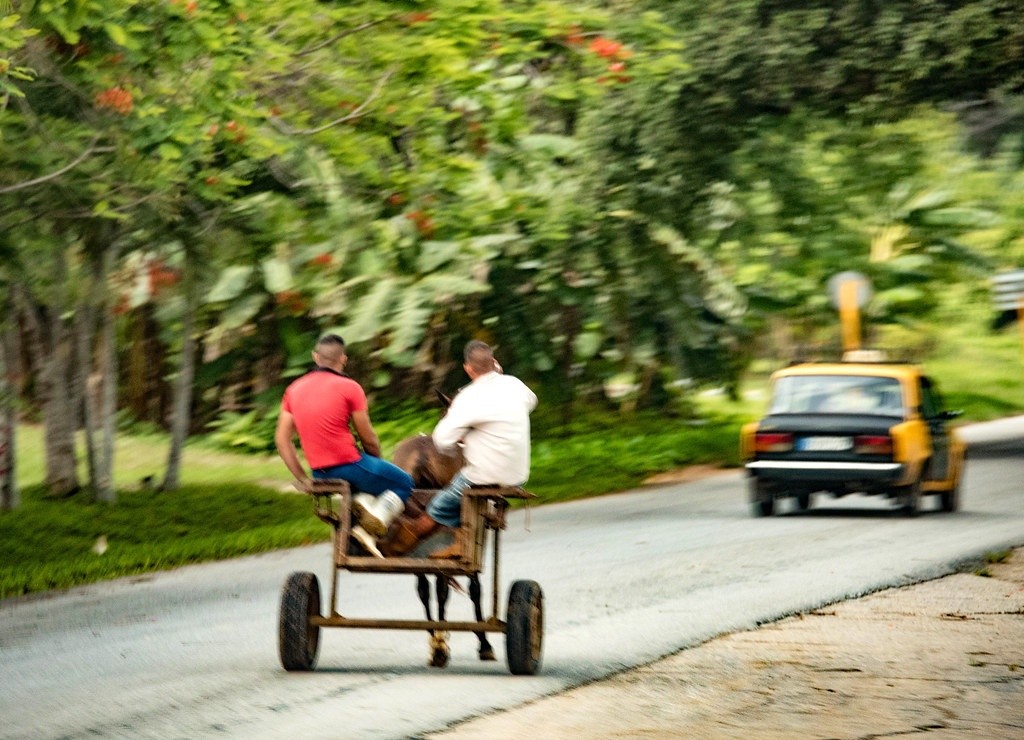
[275,334,413,559]
[377,340,538,559]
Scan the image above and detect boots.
[351,491,389,538]
[427,528,462,558]
[348,490,405,560]
[390,514,439,549]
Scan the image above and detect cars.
[738,362,968,508]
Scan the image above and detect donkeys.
[392,390,497,669]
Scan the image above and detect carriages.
[281,384,549,681]
